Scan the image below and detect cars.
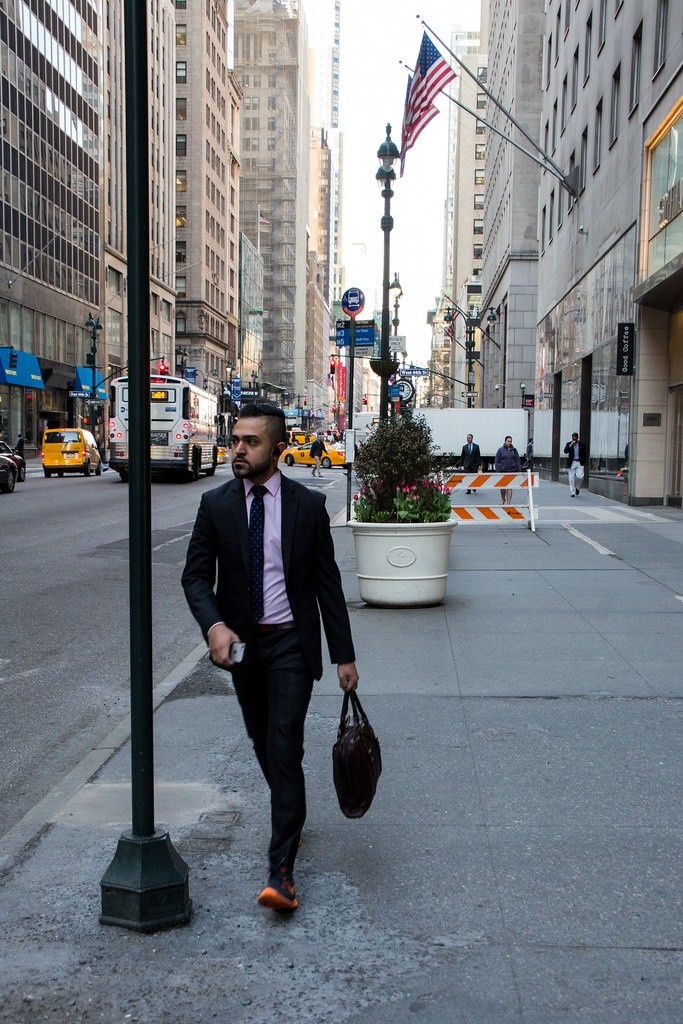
[216,447,228,463]
[0,441,27,494]
[282,441,346,468]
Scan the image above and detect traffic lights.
[159,363,166,372]
[329,360,335,374]
[362,398,368,405]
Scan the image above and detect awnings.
[0,347,46,390]
[74,366,107,400]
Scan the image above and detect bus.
[107,374,220,482]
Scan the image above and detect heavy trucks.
[351,409,531,477]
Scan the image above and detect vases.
[347,517,457,605]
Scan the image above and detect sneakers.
[258,863,299,909]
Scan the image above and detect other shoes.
[103,467,109,472]
[466,491,471,494]
[575,488,579,495]
[571,494,575,497]
[312,473,316,477]
[318,475,323,477]
[473,489,476,492]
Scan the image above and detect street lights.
[224,361,236,450]
[388,271,409,426]
[444,306,497,410]
[193,368,218,393]
[370,123,401,427]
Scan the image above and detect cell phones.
[230,642,246,662]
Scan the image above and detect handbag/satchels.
[522,461,529,469]
[333,688,382,818]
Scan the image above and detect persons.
[460,434,480,495]
[564,433,586,497]
[93,433,109,472]
[526,438,534,472]
[495,435,522,505]
[310,434,329,478]
[15,434,25,459]
[182,404,360,909]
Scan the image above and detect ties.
[469,444,471,453]
[249,486,268,623]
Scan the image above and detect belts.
[573,459,580,461]
[252,621,296,631]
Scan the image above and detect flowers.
[352,407,451,523]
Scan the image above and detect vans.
[41,428,102,478]
[286,428,342,446]
